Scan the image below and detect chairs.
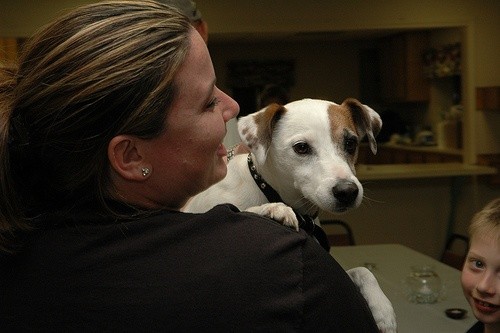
[438,233,472,269]
[318,218,359,249]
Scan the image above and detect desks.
[343,160,498,247]
[327,243,480,333]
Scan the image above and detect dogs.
[177,96,398,333]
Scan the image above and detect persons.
[462,198,500,332]
[0,1,382,333]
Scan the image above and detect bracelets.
[227,145,239,162]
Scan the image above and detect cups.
[408,268,440,305]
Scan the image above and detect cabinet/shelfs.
[356,29,430,105]
